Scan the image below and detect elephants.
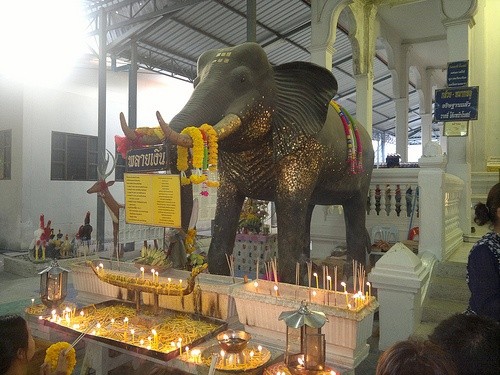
[119,42,376,286]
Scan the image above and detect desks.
[44,302,347,375]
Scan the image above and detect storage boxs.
[233,233,279,278]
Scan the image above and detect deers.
[87,149,158,261]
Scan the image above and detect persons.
[375,182,500,375]
[0,314,68,375]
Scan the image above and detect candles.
[30,262,372,375]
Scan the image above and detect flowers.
[134,124,221,188]
[239,198,270,234]
